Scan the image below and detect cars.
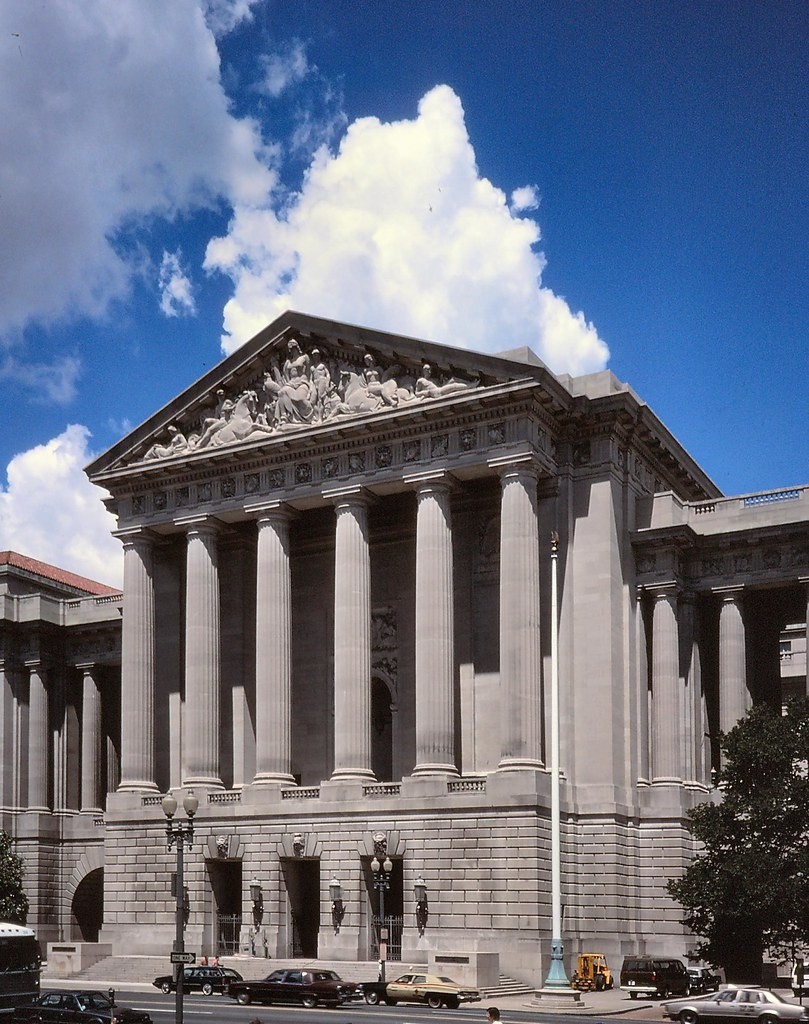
[227,968,364,1010]
[686,967,722,995]
[357,974,482,1008]
[664,989,809,1024]
[13,990,153,1024]
[152,967,244,997]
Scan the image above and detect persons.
[212,956,223,968]
[486,1007,503,1024]
[139,337,480,461]
[407,977,412,982]
[200,955,208,966]
[378,959,382,981]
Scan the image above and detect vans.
[619,955,690,1001]
[791,962,809,998]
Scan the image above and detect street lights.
[371,856,393,981]
[527,532,589,1009]
[162,790,199,1024]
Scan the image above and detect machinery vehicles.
[570,954,614,992]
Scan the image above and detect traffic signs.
[170,952,196,964]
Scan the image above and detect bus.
[0,922,45,1024]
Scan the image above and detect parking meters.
[108,988,117,1024]
